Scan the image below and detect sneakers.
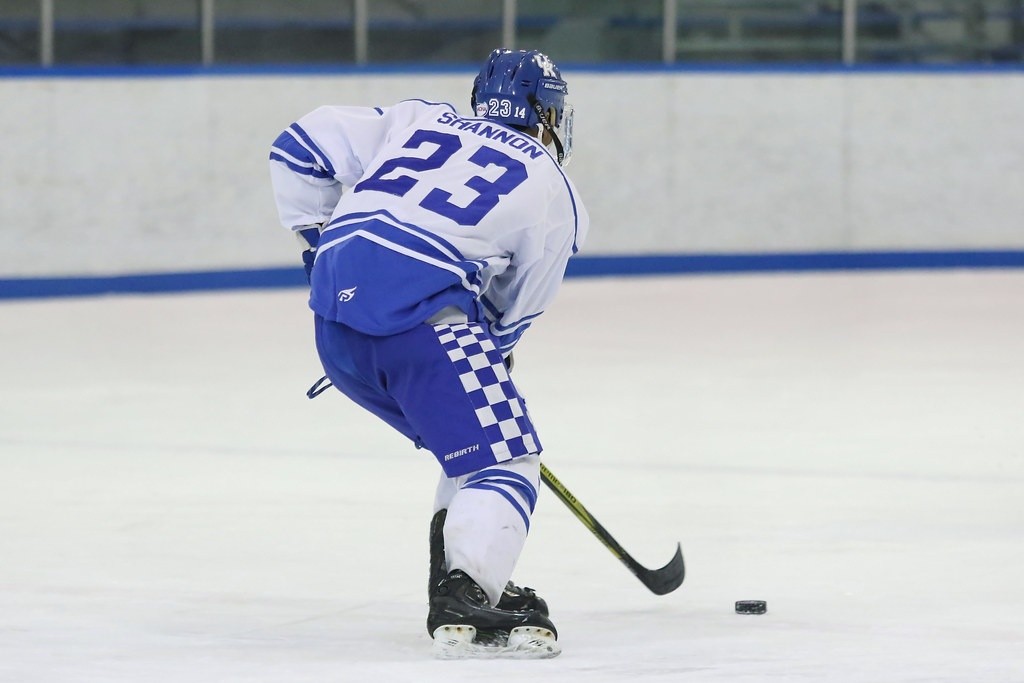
[427,566,561,659]
[427,510,550,616]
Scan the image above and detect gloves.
[301,250,317,285]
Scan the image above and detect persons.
[269,48,588,660]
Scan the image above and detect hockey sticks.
[539,460,688,598]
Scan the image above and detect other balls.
[734,599,768,615]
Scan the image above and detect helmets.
[471,46,569,129]
